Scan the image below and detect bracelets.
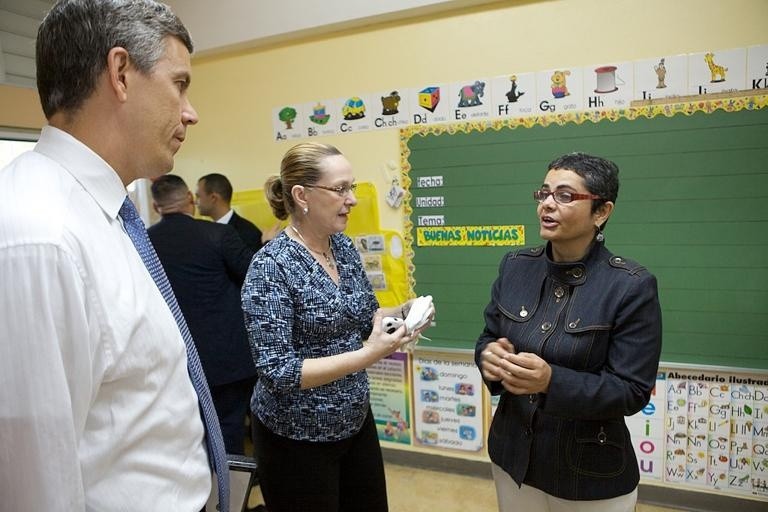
[399,304,407,323]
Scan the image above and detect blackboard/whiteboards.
[399,89,768,375]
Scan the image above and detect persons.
[469,151,666,512]
[194,171,282,258]
[139,173,284,457]
[239,139,438,511]
[0,0,213,512]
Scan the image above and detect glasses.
[533,189,601,206]
[303,182,358,196]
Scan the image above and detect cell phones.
[382,316,404,334]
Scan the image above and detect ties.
[118,196,230,511]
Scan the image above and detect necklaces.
[289,223,338,272]
